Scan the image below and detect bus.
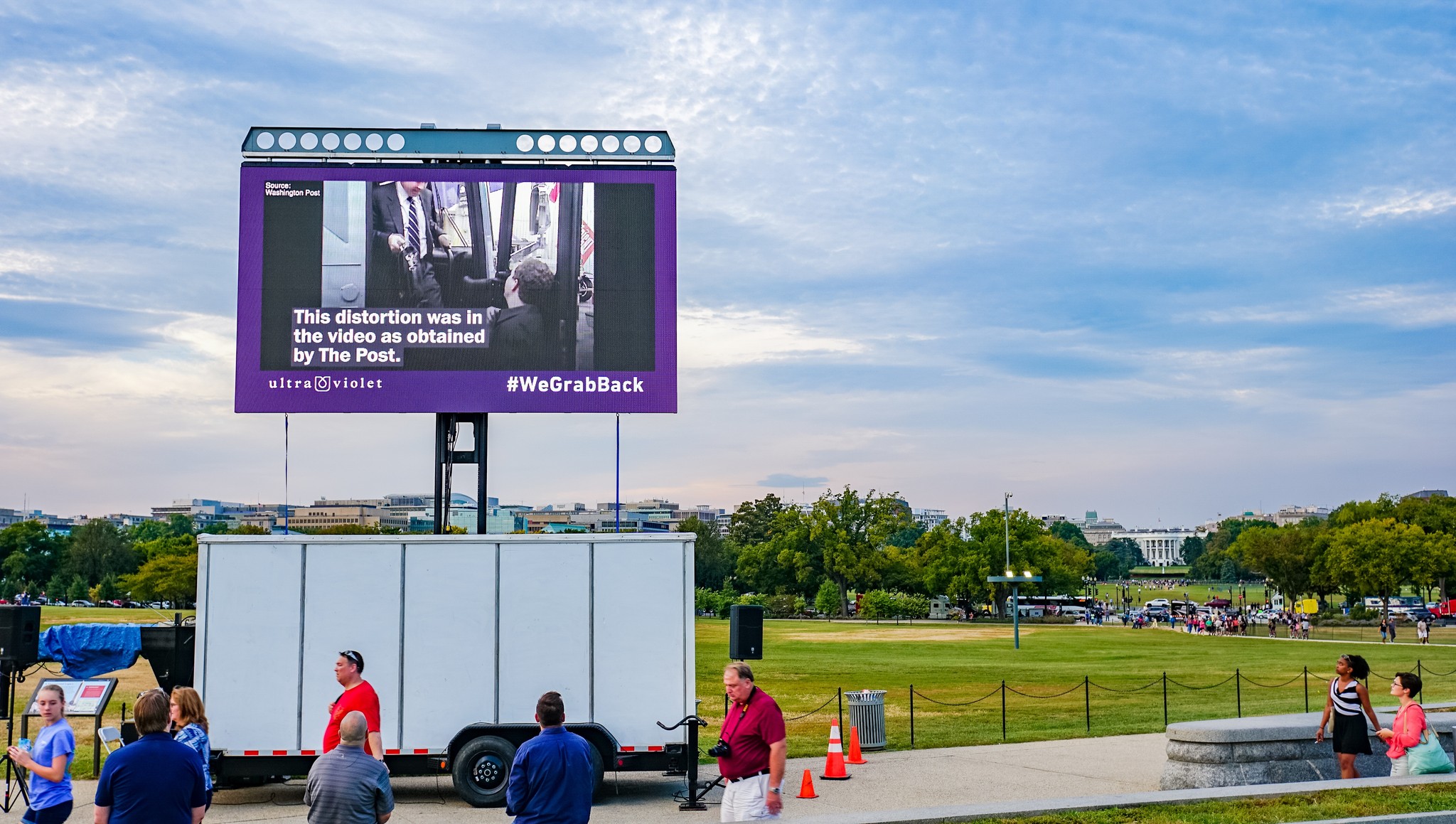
[1007,596,1093,617]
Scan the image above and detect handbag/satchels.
[1404,704,1455,775]
[1327,706,1335,734]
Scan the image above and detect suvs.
[1054,609,1086,621]
[72,600,95,607]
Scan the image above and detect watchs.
[769,787,781,794]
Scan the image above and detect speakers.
[729,604,763,660]
[0,604,41,664]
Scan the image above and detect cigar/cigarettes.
[1315,739,1321,743]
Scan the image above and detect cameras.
[708,744,731,758]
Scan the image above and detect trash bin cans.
[846,689,886,750]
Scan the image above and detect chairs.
[98,727,125,754]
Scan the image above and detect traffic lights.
[1095,588,1099,596]
[1095,598,1098,603]
[1122,589,1125,598]
[1088,587,1092,595]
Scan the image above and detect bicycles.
[1240,629,1247,636]
[1269,626,1309,640]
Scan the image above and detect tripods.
[0,664,29,813]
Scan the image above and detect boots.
[1382,638,1386,643]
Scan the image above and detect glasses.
[140,688,165,699]
[1393,680,1406,688]
[1340,654,1351,662]
[344,650,358,661]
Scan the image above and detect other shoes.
[1389,639,1392,643]
[1425,643,1429,644]
[1420,642,1424,645]
[1392,642,1394,643]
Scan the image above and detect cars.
[701,608,714,613]
[1256,609,1288,622]
[121,601,146,609]
[1117,598,1244,621]
[1317,599,1456,623]
[0,594,69,606]
[98,601,121,608]
[149,602,169,609]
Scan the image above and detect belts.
[730,768,770,784]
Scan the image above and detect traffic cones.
[844,726,869,764]
[819,717,854,780]
[796,770,819,799]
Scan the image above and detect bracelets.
[1319,726,1324,729]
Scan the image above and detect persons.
[717,661,786,822]
[367,182,562,370]
[955,609,1029,622]
[1077,579,1436,779]
[508,691,594,824]
[6,651,396,824]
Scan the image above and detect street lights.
[1266,600,1269,604]
[1114,583,1119,610]
[1264,583,1268,607]
[1082,575,1097,605]
[1238,583,1242,606]
[1266,577,1273,603]
